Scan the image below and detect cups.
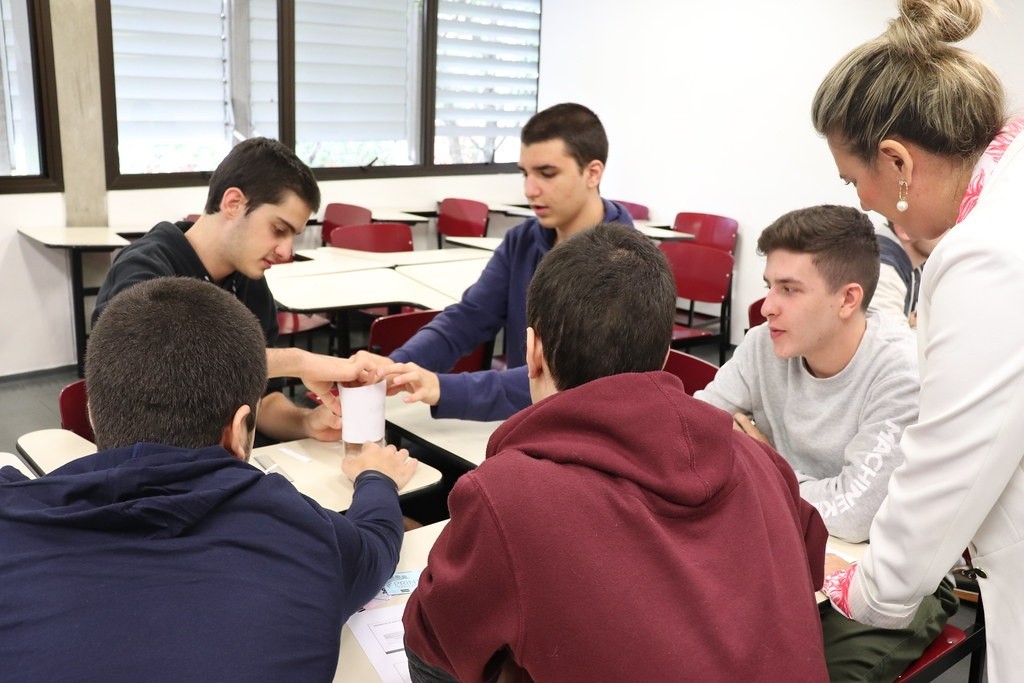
[335,377,389,444]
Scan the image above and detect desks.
[265,267,457,358]
[16,428,97,477]
[507,210,536,218]
[370,210,429,226]
[486,203,524,213]
[246,437,443,514]
[444,235,504,252]
[16,225,153,379]
[318,246,493,265]
[0,452,37,480]
[384,391,505,468]
[634,218,696,242]
[330,518,451,683]
[265,247,397,280]
[395,257,491,302]
[402,208,443,250]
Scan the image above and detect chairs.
[63,198,986,683]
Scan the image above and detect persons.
[0,276,417,683]
[354,99,665,524]
[86,137,363,444]
[400,222,831,683]
[855,198,958,340]
[803,1,1024,683]
[690,198,961,682]
